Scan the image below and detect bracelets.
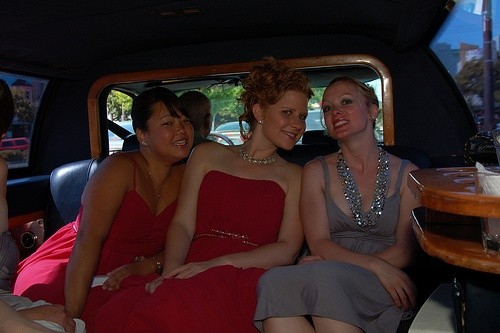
[151,255,162,275]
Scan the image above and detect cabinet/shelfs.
[407,165,500,333]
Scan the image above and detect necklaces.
[145,168,168,200]
[336,143,392,227]
[239,141,279,168]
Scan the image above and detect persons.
[0,79,20,289]
[252,77,424,333]
[95,58,315,333]
[0,288,87,333]
[13,86,195,318]
[179,91,213,138]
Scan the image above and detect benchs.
[42,146,434,243]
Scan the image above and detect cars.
[107,120,136,155]
[209,121,251,146]
[295,107,327,145]
[0,136,31,152]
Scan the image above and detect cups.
[474,169,500,253]
